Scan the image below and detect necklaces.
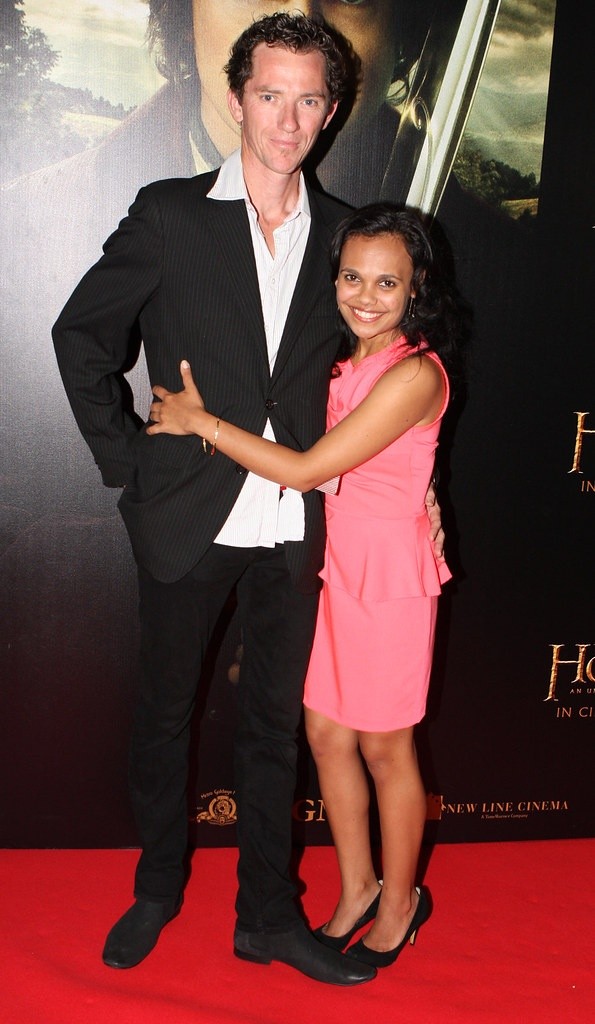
[190,111,226,171]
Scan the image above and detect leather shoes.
[102,896,182,970]
[234,922,377,986]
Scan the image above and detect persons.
[52,6,446,983]
[0,0,522,498]
[143,201,453,969]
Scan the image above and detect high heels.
[346,885,429,968]
[312,886,386,950]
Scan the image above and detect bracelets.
[203,419,219,456]
